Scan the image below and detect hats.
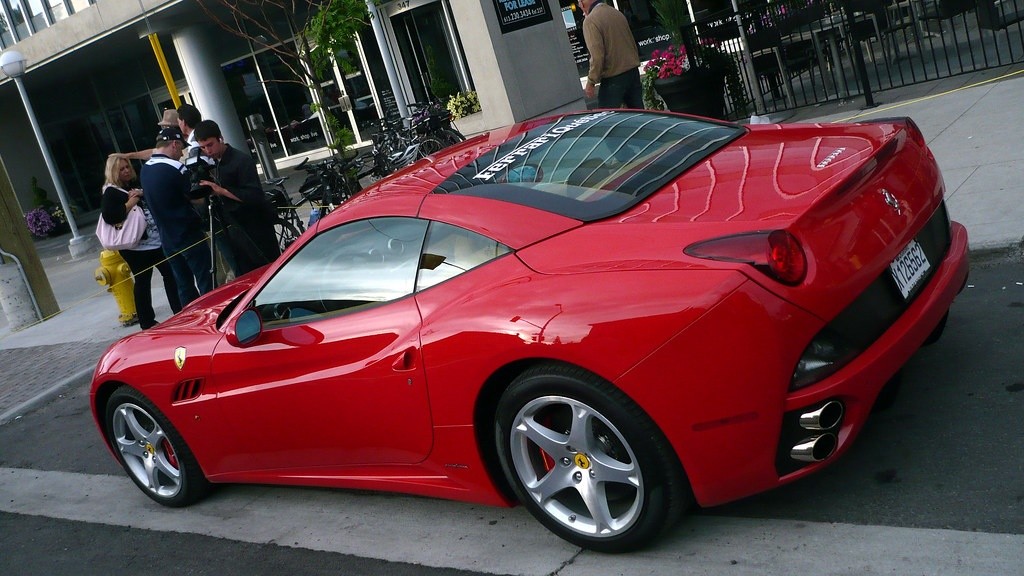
[158,109,180,125]
[156,126,189,148]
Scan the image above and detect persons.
[578,0,645,110]
[102,104,282,331]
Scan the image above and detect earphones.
[219,138,223,144]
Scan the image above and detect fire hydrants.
[95,250,139,326]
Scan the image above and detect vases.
[653,67,726,120]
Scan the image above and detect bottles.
[309,208,319,227]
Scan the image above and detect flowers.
[641,36,750,119]
[408,101,446,132]
[446,89,481,121]
[24,195,80,239]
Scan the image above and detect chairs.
[747,0,1024,102]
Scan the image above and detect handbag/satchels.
[94,184,147,252]
[188,159,213,198]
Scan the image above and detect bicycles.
[261,101,467,255]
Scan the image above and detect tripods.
[208,194,274,290]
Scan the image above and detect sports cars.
[90,111,975,553]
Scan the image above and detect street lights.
[0,50,92,259]
